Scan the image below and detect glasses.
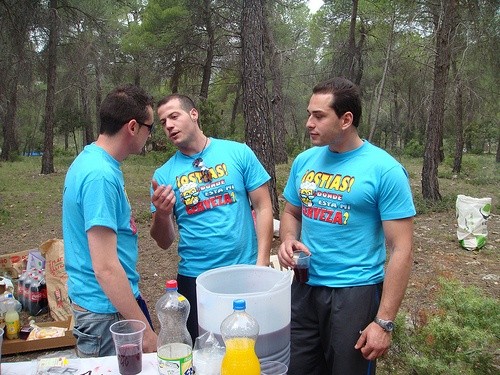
[135,120,155,133]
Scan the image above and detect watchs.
[373,317,395,333]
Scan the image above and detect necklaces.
[181,137,212,183]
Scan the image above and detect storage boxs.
[2,315,76,354]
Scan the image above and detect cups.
[292,249,311,283]
[0,328,5,363]
[109,319,146,375]
[260,361,288,375]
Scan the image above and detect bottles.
[5,294,20,340]
[220,298,261,375]
[18,268,49,317]
[156,279,194,375]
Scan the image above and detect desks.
[0,347,197,375]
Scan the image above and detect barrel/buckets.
[196,264,291,375]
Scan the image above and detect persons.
[149,95,274,349]
[278,78,417,375]
[62,84,158,358]
